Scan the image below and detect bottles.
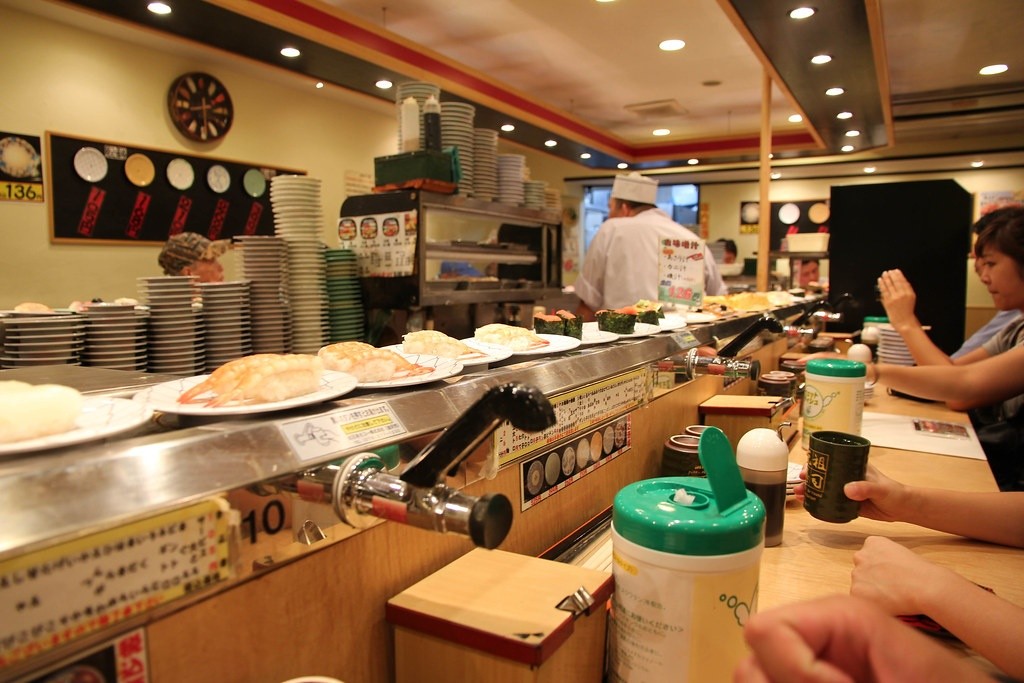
[777,328,799,350]
[756,359,805,403]
[802,360,866,445]
[861,317,889,359]
[608,426,767,682]
[400,96,419,151]
[660,434,707,478]
[685,425,724,437]
[422,93,442,150]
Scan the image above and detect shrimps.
[178,352,323,408]
[318,342,432,383]
[474,323,548,351]
[401,331,482,359]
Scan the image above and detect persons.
[572,171,728,324]
[729,204,1024,683]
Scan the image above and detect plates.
[131,370,357,414]
[875,324,917,366]
[0,148,364,370]
[580,311,716,343]
[356,353,463,387]
[0,137,37,178]
[460,334,582,355]
[394,80,562,215]
[383,341,512,366]
[0,396,154,453]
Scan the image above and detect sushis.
[0,298,664,446]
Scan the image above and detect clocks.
[168,70,235,142]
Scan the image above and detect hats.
[611,172,660,206]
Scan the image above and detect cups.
[803,430,871,523]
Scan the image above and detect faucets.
[831,292,853,305]
[398,381,557,490]
[716,316,784,358]
[791,300,831,327]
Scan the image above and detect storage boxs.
[699,394,784,453]
[783,398,801,444]
[386,545,615,683]
[374,151,453,186]
[787,232,829,252]
